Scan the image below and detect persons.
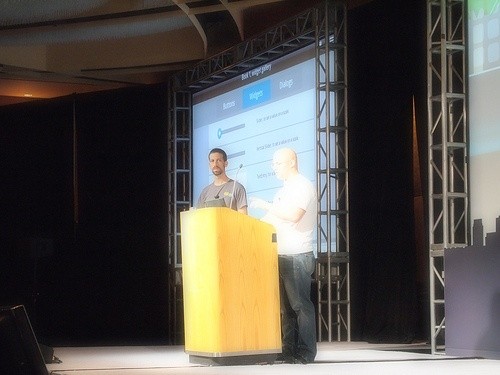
[262,147,322,363]
[190,147,251,219]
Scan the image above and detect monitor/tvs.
[204,198,226,208]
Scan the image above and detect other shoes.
[276,346,297,360]
[287,350,314,364]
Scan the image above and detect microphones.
[229,163,243,209]
